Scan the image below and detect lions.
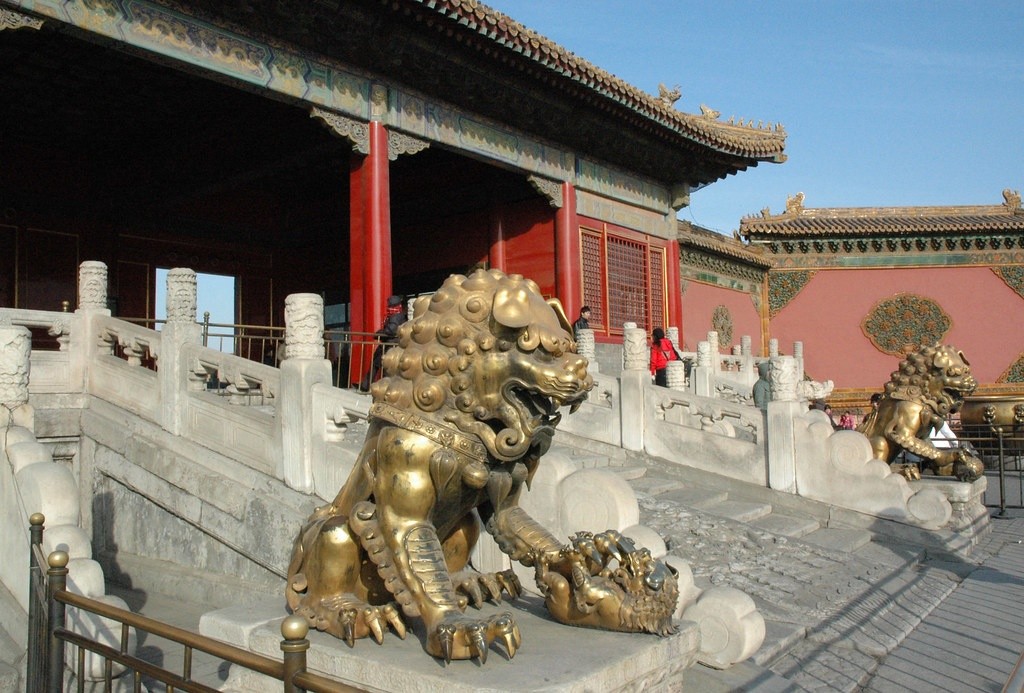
[854,340,979,481]
[286,266,680,666]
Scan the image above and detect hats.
[824,404,831,411]
[388,296,401,306]
[870,393,881,402]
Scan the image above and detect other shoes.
[352,383,369,392]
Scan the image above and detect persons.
[753,363,772,409]
[573,306,591,342]
[263,345,280,367]
[352,296,405,391]
[323,328,336,368]
[804,370,960,476]
[650,328,678,387]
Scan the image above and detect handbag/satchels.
[676,356,686,364]
